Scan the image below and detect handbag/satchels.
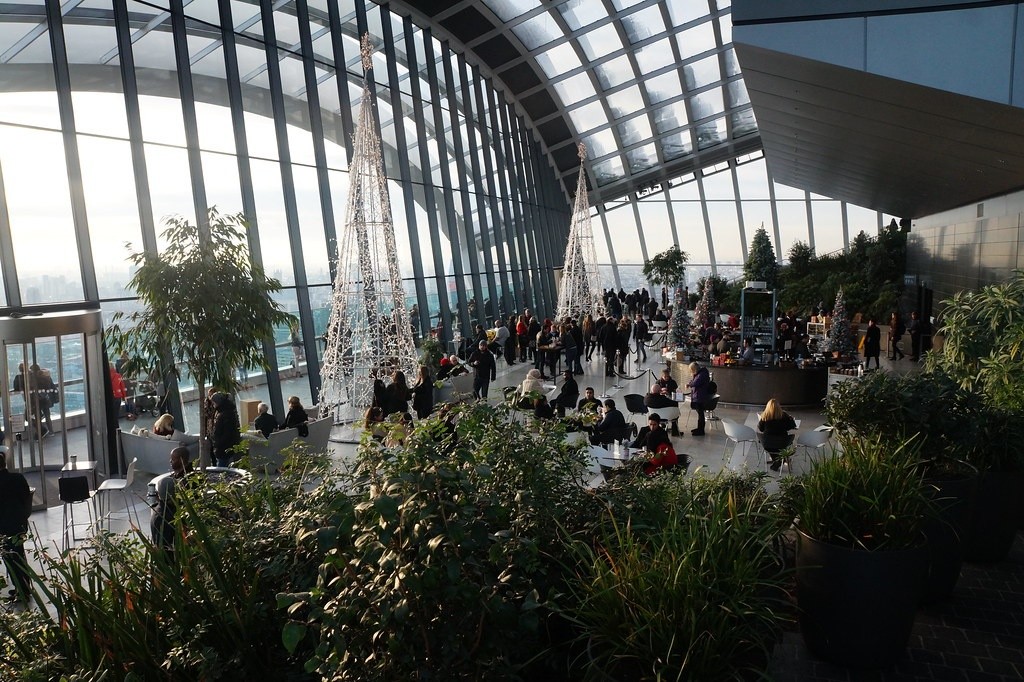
[591,335,597,342]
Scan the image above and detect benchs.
[121,432,199,476]
[347,357,475,409]
[240,412,334,474]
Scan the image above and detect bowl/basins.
[734,359,746,365]
[724,363,735,367]
[752,364,756,366]
[745,361,752,366]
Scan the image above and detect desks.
[757,420,801,468]
[60,461,98,540]
[602,445,642,460]
[664,394,685,431]
[538,342,566,385]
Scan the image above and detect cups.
[557,338,559,342]
[642,446,647,451]
[672,393,675,400]
[70,456,77,463]
[836,368,858,376]
[685,385,689,391]
[147,492,157,506]
[147,484,155,495]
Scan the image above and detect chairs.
[652,320,667,330]
[502,371,833,484]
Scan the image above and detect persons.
[534,395,556,433]
[685,361,711,436]
[365,366,457,455]
[643,370,684,437]
[13,363,59,441]
[0,450,33,604]
[203,387,244,469]
[630,413,677,479]
[151,446,200,546]
[155,360,169,416]
[378,287,670,402]
[592,399,626,448]
[578,386,603,417]
[280,396,309,430]
[109,361,128,428]
[254,403,277,438]
[699,309,923,368]
[758,400,796,470]
[115,350,137,420]
[153,413,175,436]
[289,327,302,378]
[549,371,579,418]
[676,284,690,309]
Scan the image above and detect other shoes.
[691,429,705,436]
[671,432,684,436]
[4,590,31,604]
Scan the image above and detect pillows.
[247,405,320,439]
[131,424,199,445]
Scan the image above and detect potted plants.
[100,206,305,514]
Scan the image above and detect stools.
[57,456,138,555]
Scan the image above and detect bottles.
[744,313,773,327]
[762,348,781,364]
[743,328,773,340]
[857,364,863,377]
[783,354,787,361]
[598,442,614,451]
[795,354,803,362]
